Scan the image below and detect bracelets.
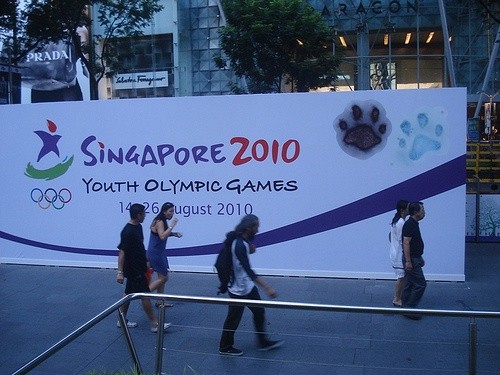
[169,227,172,229]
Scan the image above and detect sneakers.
[150,322,172,331]
[116,319,137,327]
[219,348,243,355]
[258,339,284,351]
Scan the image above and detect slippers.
[392,302,402,307]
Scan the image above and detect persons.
[401,201,426,320]
[20,21,90,104]
[116,203,172,331]
[143,201,183,307]
[390,199,413,307]
[217,214,286,356]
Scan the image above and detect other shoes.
[155,300,174,308]
[400,313,422,320]
